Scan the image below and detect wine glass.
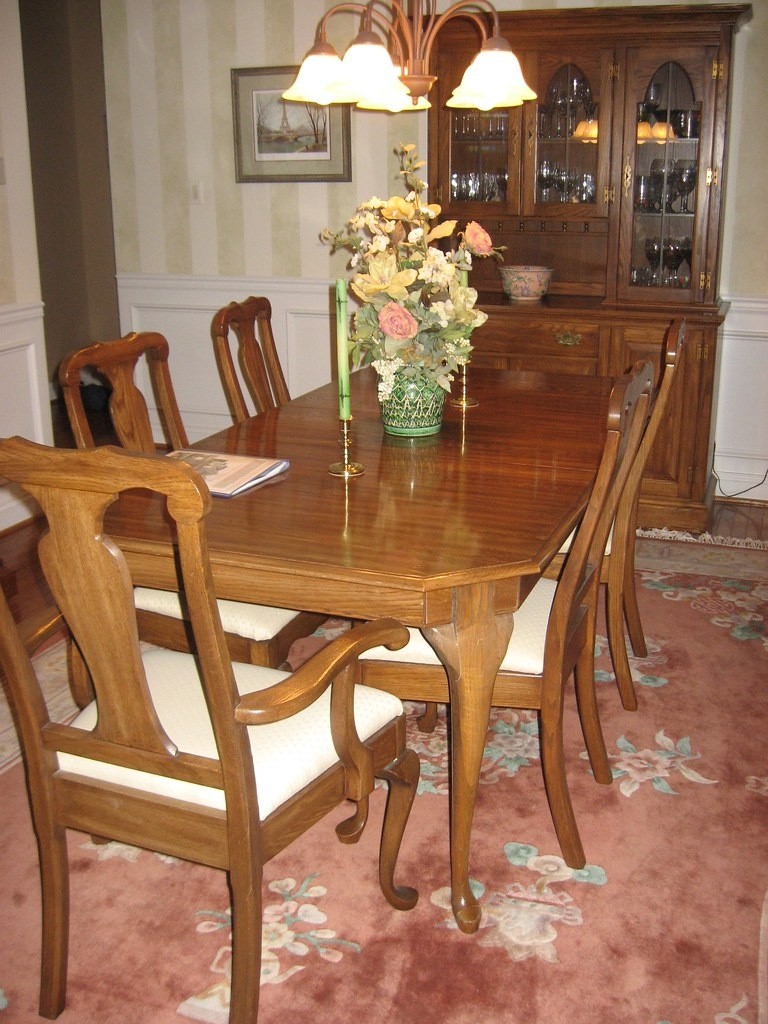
[650,158,698,214]
[631,236,693,288]
[538,76,598,139]
[635,82,702,138]
[537,160,596,203]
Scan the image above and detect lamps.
[569,114,676,144]
[281,1,536,114]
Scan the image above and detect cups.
[453,109,508,141]
[452,169,508,202]
[635,175,652,213]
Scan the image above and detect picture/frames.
[229,65,354,185]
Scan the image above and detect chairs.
[334,359,657,867]
[0,435,420,1023]
[211,294,295,427]
[549,316,689,714]
[59,330,329,709]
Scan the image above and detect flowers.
[319,140,508,402]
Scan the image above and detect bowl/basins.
[497,265,554,301]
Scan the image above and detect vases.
[493,262,554,302]
[375,360,446,437]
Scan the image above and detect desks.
[103,366,628,931]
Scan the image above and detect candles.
[334,278,351,418]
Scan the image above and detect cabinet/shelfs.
[410,2,754,534]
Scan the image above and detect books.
[151,449,291,498]
